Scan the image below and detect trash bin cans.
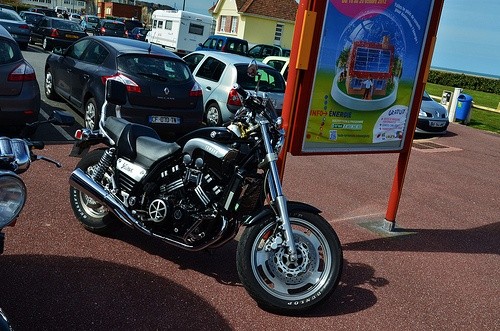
[464,101,475,124]
[440,90,453,111]
[455,94,473,120]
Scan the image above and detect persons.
[79,10,85,15]
[57,11,63,17]
[62,10,69,19]
[362,77,373,99]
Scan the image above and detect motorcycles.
[0,109,76,331]
[67,60,343,312]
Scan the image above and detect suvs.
[247,43,290,59]
[194,36,249,57]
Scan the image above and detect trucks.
[145,9,213,54]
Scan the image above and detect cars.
[0,4,151,52]
[0,23,42,134]
[44,35,203,142]
[415,89,450,134]
[255,55,290,85]
[165,51,287,128]
